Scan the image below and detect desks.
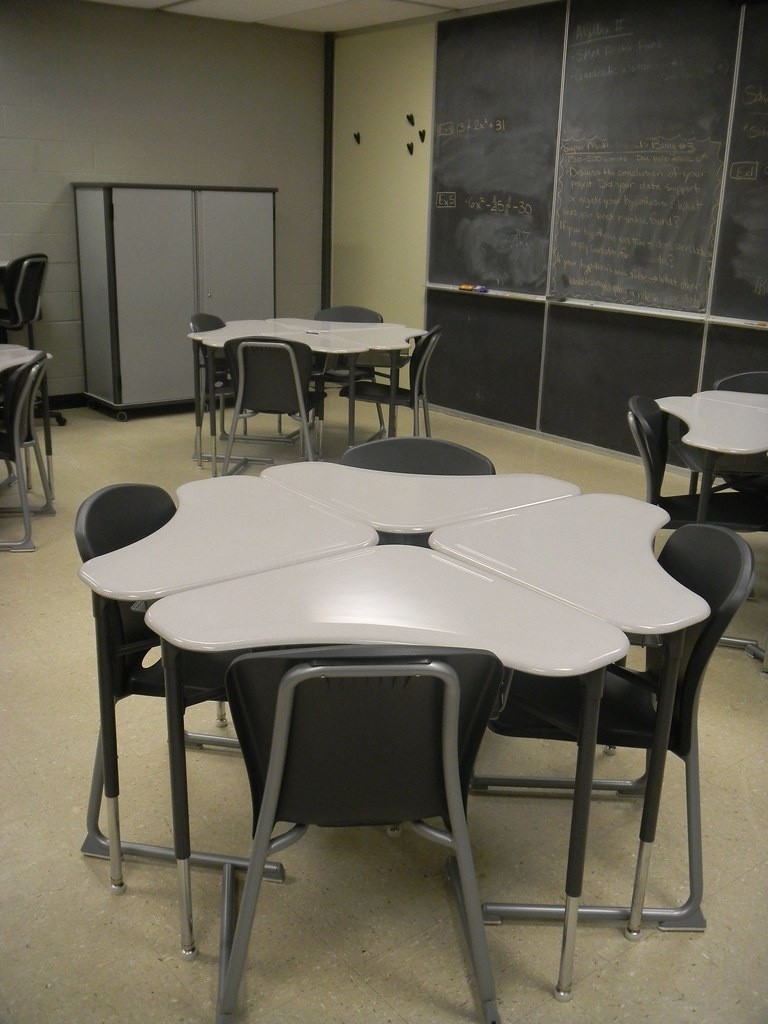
[187,317,428,479]
[0,344,54,502]
[627,389,767,676]
[76,459,712,1000]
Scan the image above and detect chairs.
[65,483,309,883]
[221,335,327,478]
[301,307,387,451]
[0,253,69,429]
[615,395,766,652]
[1,349,54,551]
[464,523,754,931]
[339,322,444,456]
[340,437,503,476]
[713,370,768,494]
[213,641,507,1024]
[187,312,294,468]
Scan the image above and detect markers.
[546,296,566,302]
[459,285,489,293]
[744,322,767,326]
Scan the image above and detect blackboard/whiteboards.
[705,1,768,328]
[538,295,708,482]
[545,1,747,323]
[698,313,768,484]
[416,283,548,437]
[426,1,569,302]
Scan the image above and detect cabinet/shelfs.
[71,182,281,426]
